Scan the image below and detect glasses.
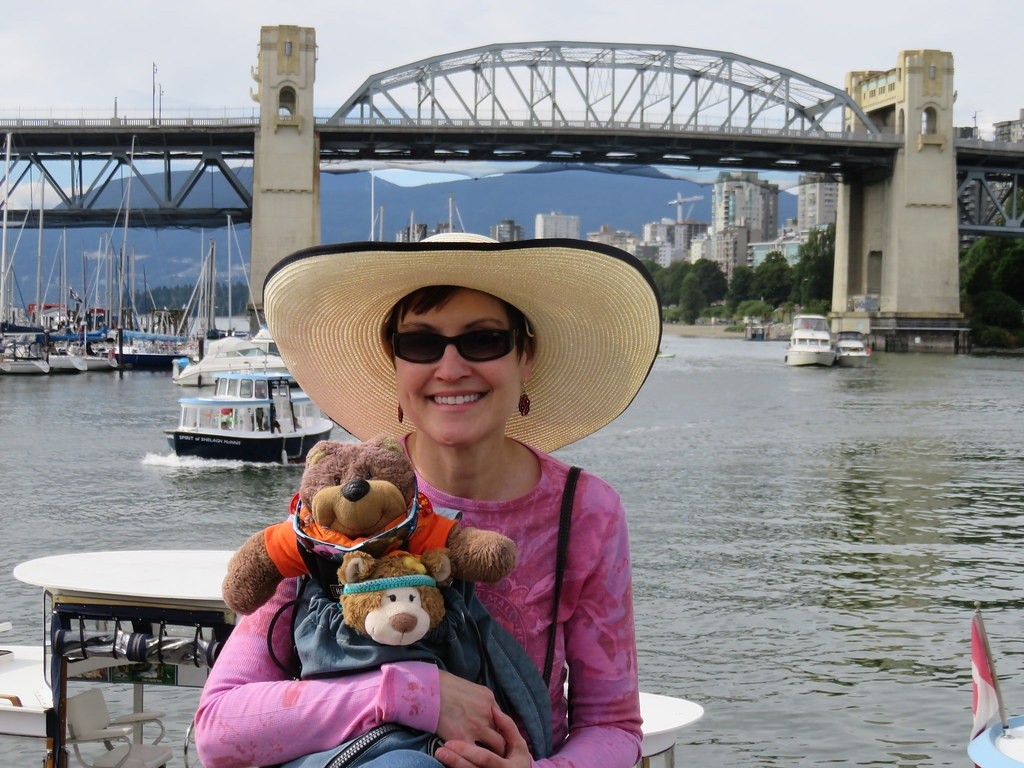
[391,328,516,364]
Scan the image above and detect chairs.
[66,687,173,768]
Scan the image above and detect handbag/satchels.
[282,576,553,768]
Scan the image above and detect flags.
[970,615,999,768]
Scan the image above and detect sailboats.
[0,132,238,374]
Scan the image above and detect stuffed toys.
[222,434,516,645]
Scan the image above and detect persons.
[194,232,663,768]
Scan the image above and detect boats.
[162,370,335,466]
[837,338,869,367]
[784,314,837,368]
[172,335,297,385]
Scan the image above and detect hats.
[262,234,663,455]
